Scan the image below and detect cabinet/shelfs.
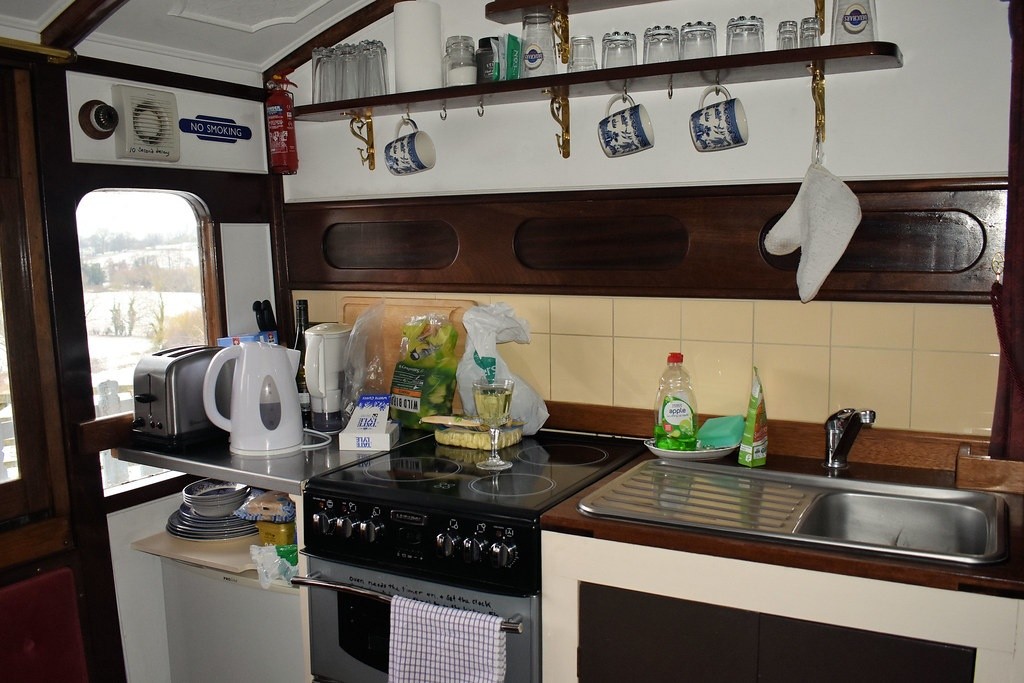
[540,523,1024,683]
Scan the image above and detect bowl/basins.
[183,477,251,515]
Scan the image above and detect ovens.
[292,556,542,683]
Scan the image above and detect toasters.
[130,345,236,444]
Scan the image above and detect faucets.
[823,407,876,470]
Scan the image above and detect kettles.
[203,341,303,456]
[800,17,821,48]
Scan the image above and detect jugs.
[304,322,353,415]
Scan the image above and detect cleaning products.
[654,351,698,451]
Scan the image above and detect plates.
[645,438,740,461]
[166,489,268,542]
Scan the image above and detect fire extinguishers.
[265,69,300,174]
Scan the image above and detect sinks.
[792,488,1009,565]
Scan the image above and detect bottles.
[444,36,477,87]
[476,37,500,84]
[654,353,698,451]
[520,10,558,79]
[292,300,311,417]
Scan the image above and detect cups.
[680,21,717,60]
[567,35,597,74]
[597,94,654,158]
[689,84,749,152]
[311,40,389,104]
[777,21,798,50]
[726,16,765,56]
[602,31,637,69]
[384,119,436,176]
[643,26,679,64]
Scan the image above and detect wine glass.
[473,378,513,470]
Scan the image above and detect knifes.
[252,300,280,344]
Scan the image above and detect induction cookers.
[303,429,639,595]
